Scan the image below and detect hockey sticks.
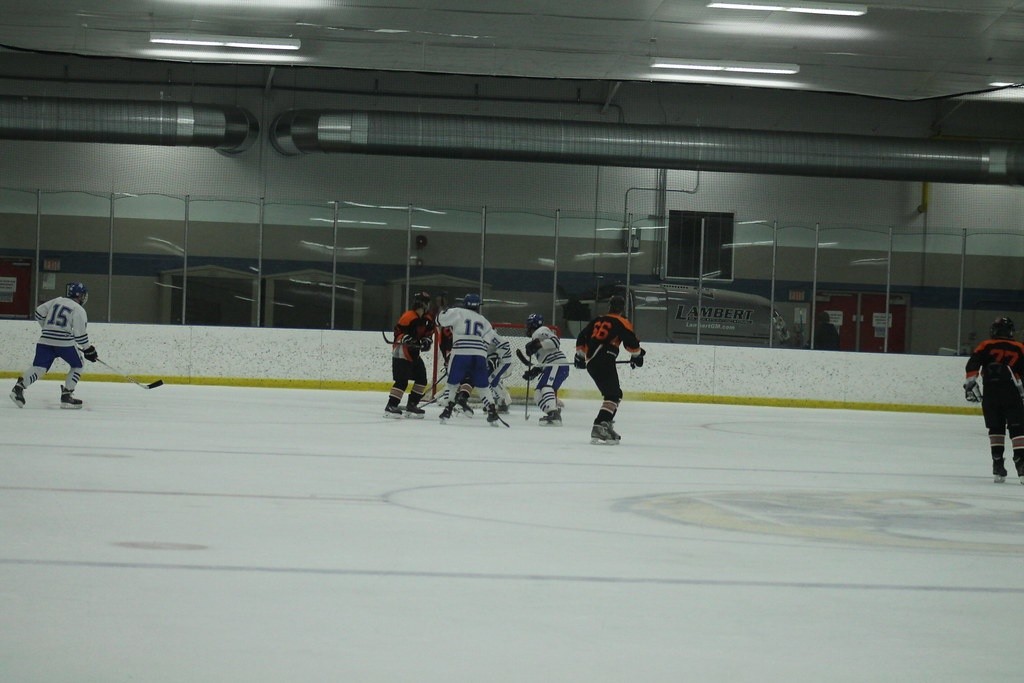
[381,328,425,348]
[497,414,510,427]
[78,346,164,390]
[516,348,631,366]
[409,372,446,399]
[525,355,532,420]
[399,393,444,410]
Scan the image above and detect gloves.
[82,345,98,363]
[420,336,433,353]
[524,339,541,357]
[573,353,587,370]
[521,366,542,381]
[630,347,646,369]
[401,334,416,345]
[963,381,982,402]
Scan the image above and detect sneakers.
[59,384,83,409]
[403,393,425,419]
[438,402,456,424]
[9,377,26,409]
[590,420,621,446]
[485,403,498,426]
[1012,455,1024,485]
[991,453,1008,483]
[539,406,562,427]
[383,401,403,420]
[482,397,510,415]
[453,391,474,419]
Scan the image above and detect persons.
[574,296,646,446]
[963,317,1024,483]
[805,312,840,350]
[961,330,980,355]
[434,294,499,427]
[483,334,515,414]
[9,281,99,410]
[383,290,435,419]
[522,313,570,427]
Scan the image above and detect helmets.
[64,280,89,307]
[606,295,626,314]
[988,316,1014,339]
[412,291,432,307]
[525,312,545,338]
[462,292,480,313]
